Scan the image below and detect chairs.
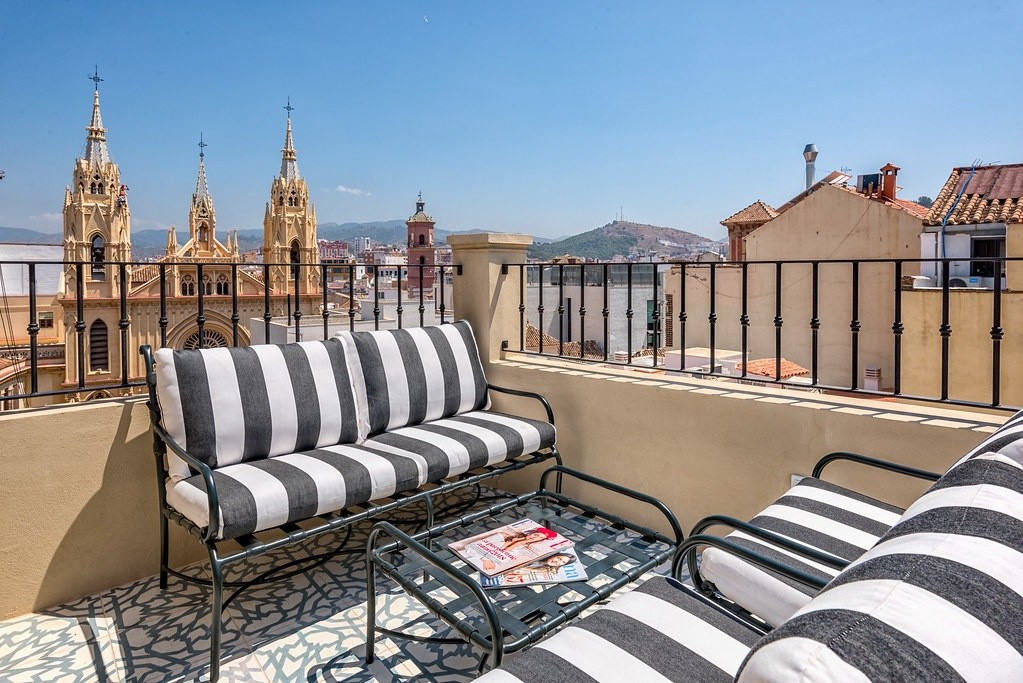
[468,409,1022,683]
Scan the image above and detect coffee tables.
[366,465,685,678]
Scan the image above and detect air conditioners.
[949,276,982,287]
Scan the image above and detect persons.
[457,526,556,571]
[501,551,577,584]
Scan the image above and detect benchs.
[139,319,563,683]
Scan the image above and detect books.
[447,517,576,578]
[477,544,588,589]
